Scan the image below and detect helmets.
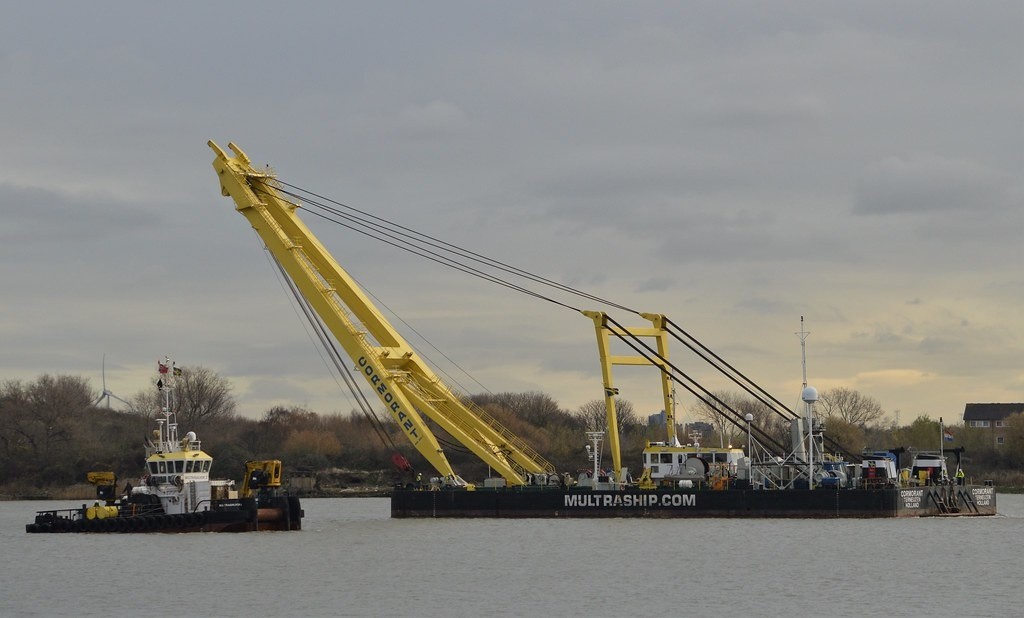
[959,469,961,471]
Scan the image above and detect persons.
[940,468,947,477]
[416,473,423,491]
[955,469,964,477]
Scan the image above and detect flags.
[159,363,167,373]
[943,430,954,442]
[157,379,163,391]
[173,367,182,377]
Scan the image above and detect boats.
[25,358,305,533]
[205,139,998,520]
